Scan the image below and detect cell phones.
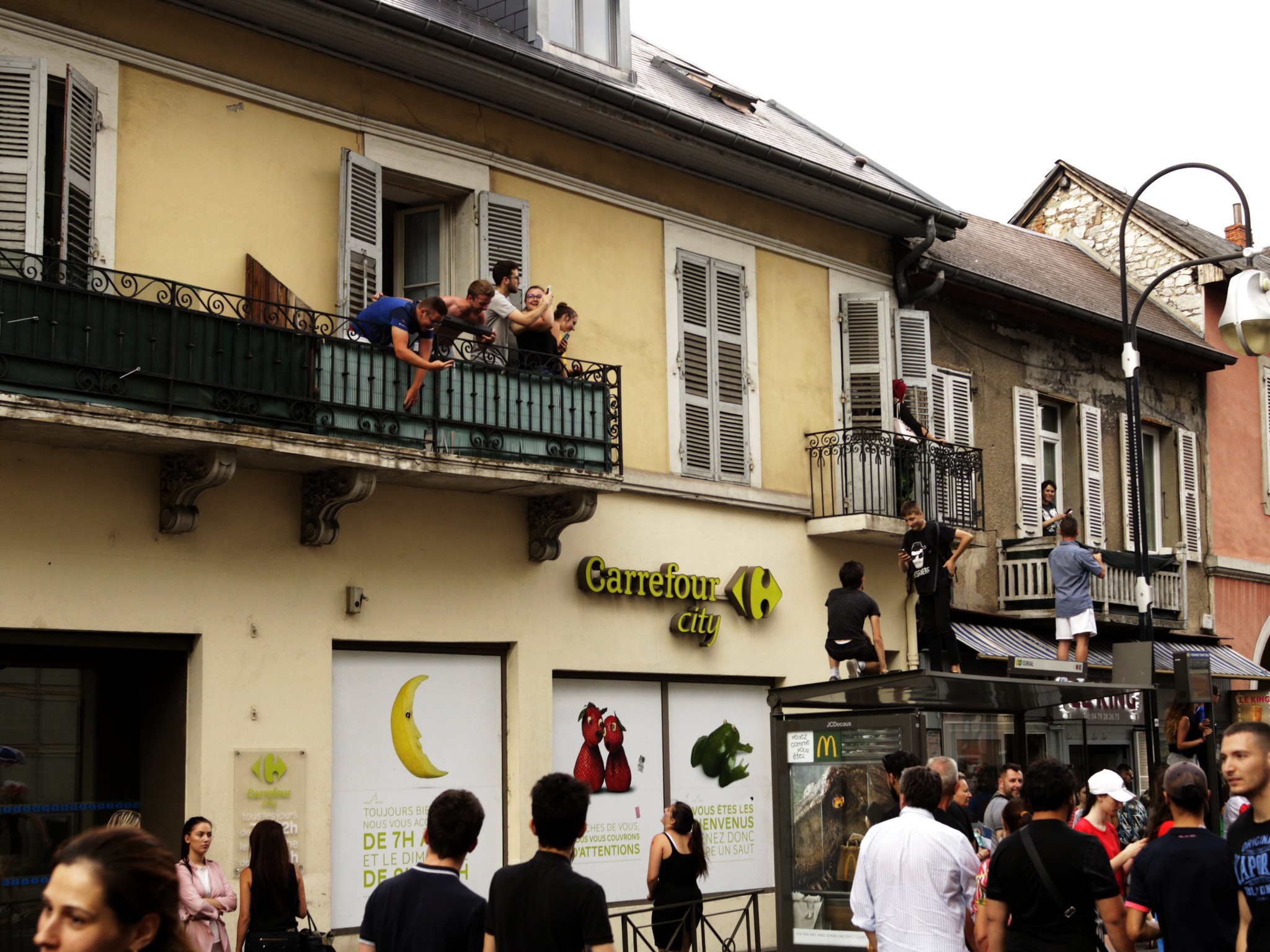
[1065,508,1072,514]
[1142,830,1149,839]
[558,332,570,348]
[546,285,551,295]
[1093,548,1101,554]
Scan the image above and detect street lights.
[1118,162,1270,799]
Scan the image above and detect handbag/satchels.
[836,840,860,881]
[298,911,336,952]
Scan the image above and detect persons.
[33,772,614,952]
[1048,517,1106,683]
[348,260,578,410]
[873,378,946,517]
[897,500,973,674]
[850,686,1270,952]
[1041,480,1073,536]
[824,560,889,681]
[646,801,709,952]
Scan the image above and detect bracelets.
[932,435,934,440]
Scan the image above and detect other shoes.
[846,659,861,678]
[829,677,838,681]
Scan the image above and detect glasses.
[508,276,519,279]
[1126,773,1134,780]
[525,294,542,300]
[426,311,443,327]
[1216,694,1222,697]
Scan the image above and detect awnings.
[949,621,1270,681]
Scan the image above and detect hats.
[1164,761,1207,798]
[1088,769,1137,802]
